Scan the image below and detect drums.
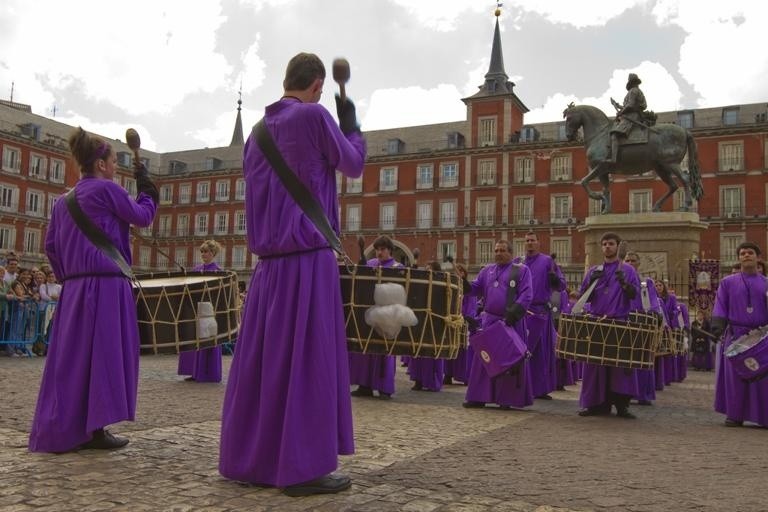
[554,311,688,369]
[724,323,768,383]
[339,264,471,361]
[469,320,527,377]
[129,270,242,356]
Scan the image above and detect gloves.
[589,270,603,285]
[711,329,722,343]
[506,303,525,326]
[335,93,360,132]
[132,157,155,188]
[550,272,560,289]
[615,270,626,286]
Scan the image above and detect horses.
[562,101,705,215]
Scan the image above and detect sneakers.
[725,418,743,427]
[10,353,29,358]
[638,399,652,406]
[285,471,352,496]
[579,407,636,420]
[462,400,511,410]
[534,395,552,400]
[443,375,452,384]
[31,347,43,356]
[351,386,390,399]
[84,429,129,450]
[411,381,423,390]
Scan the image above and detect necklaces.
[280,95,304,104]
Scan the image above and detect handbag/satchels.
[694,341,706,353]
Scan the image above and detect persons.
[0,255,65,357]
[602,73,647,164]
[27,126,160,454]
[217,51,367,497]
[571,233,689,421]
[344,234,404,401]
[401,230,589,411]
[176,240,223,384]
[690,242,767,429]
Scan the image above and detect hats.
[39,263,51,271]
